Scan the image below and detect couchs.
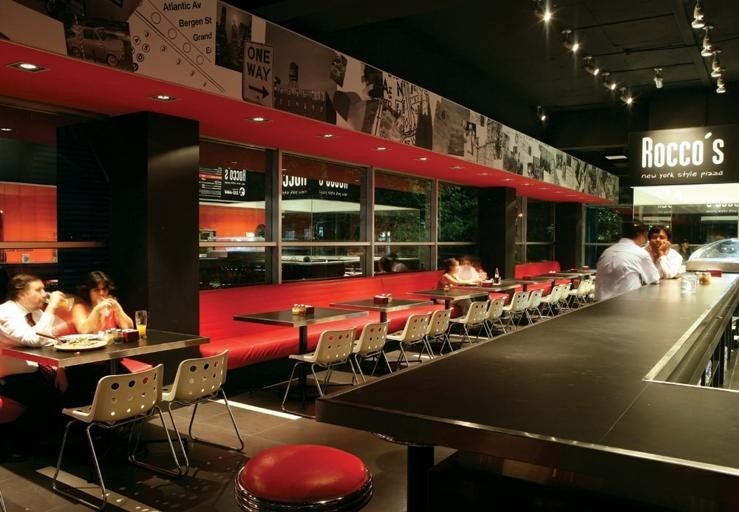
[514,261,580,297]
[195,270,448,395]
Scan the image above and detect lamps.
[716,69,726,93]
[710,49,724,78]
[583,55,599,76]
[691,1,705,29]
[654,68,663,88]
[602,73,616,90]
[701,25,713,57]
[620,87,632,104]
[560,30,579,53]
[535,0,556,24]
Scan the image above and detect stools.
[233,444,375,511]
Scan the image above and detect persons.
[441,258,481,314]
[0,274,70,459]
[456,257,480,279]
[715,228,727,240]
[643,223,684,280]
[594,218,660,302]
[66,271,136,405]
[246,225,266,253]
[678,237,691,261]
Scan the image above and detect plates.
[53,334,111,350]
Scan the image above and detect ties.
[26,313,36,327]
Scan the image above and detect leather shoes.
[2,447,24,462]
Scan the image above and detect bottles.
[292,304,298,314]
[300,305,305,316]
[495,267,499,284]
[444,285,449,291]
[297,305,301,314]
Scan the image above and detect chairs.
[50,363,182,509]
[460,297,506,348]
[566,280,589,309]
[323,321,392,395]
[532,286,563,319]
[399,307,453,368]
[282,326,360,419]
[491,291,531,334]
[131,349,244,475]
[440,300,490,352]
[371,311,433,376]
[548,283,572,315]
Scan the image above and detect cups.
[135,311,149,340]
